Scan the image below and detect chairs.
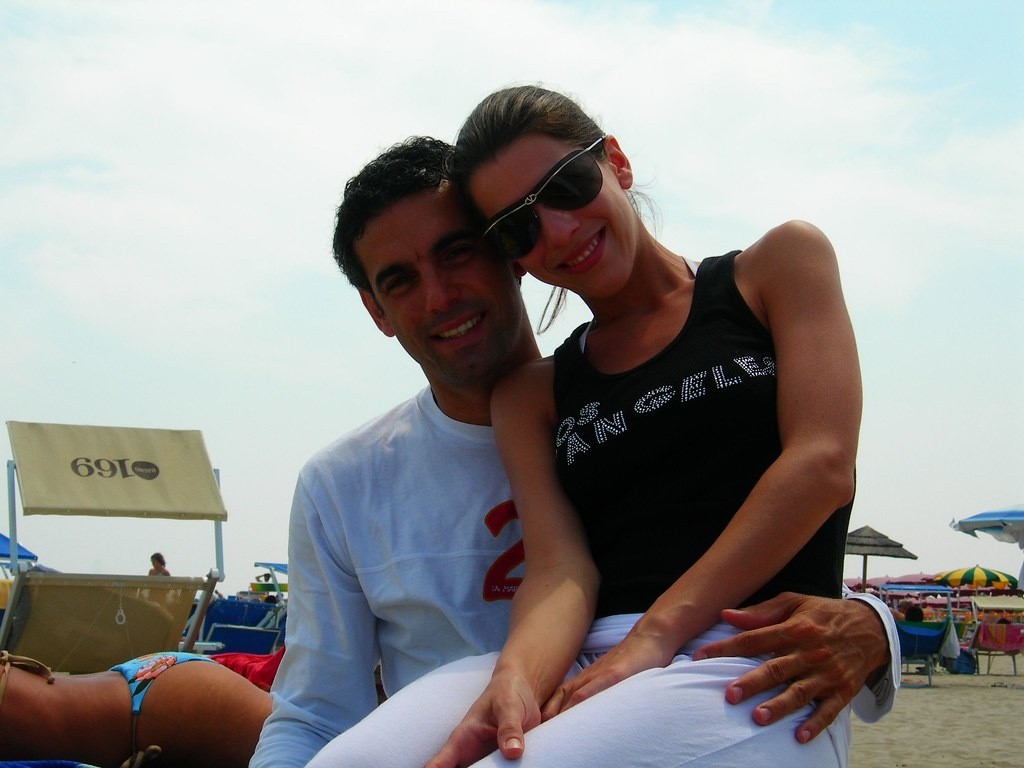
[181,596,287,657]
[895,616,1024,686]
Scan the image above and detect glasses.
[483,133,607,262]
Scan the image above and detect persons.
[959,618,1011,647]
[916,660,939,673]
[905,606,924,621]
[303,84,863,768]
[0,651,273,768]
[149,553,170,576]
[888,600,914,620]
[249,136,902,768]
[214,571,287,604]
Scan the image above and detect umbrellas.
[0,533,38,563]
[845,526,918,593]
[843,564,1018,620]
[951,510,1024,550]
